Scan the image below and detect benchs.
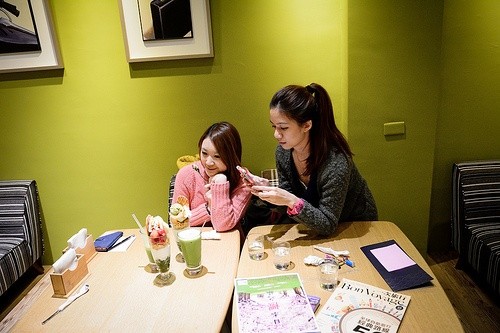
[449,160,500,308]
[0,179,45,297]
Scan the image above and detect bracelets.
[287,199,304,215]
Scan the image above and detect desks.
[8,226,241,333]
[231,219,466,333]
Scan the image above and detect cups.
[140,227,176,285]
[247,232,264,260]
[171,217,191,262]
[177,228,202,276]
[272,241,291,270]
[318,258,339,290]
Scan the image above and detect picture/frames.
[117,0,216,64]
[0,0,65,75]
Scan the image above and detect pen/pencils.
[108,235,131,250]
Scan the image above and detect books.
[307,294,321,313]
[235,273,320,333]
[316,277,411,333]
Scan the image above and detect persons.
[240,83,379,233]
[170,121,251,231]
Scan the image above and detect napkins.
[201,229,222,240]
[313,246,350,258]
